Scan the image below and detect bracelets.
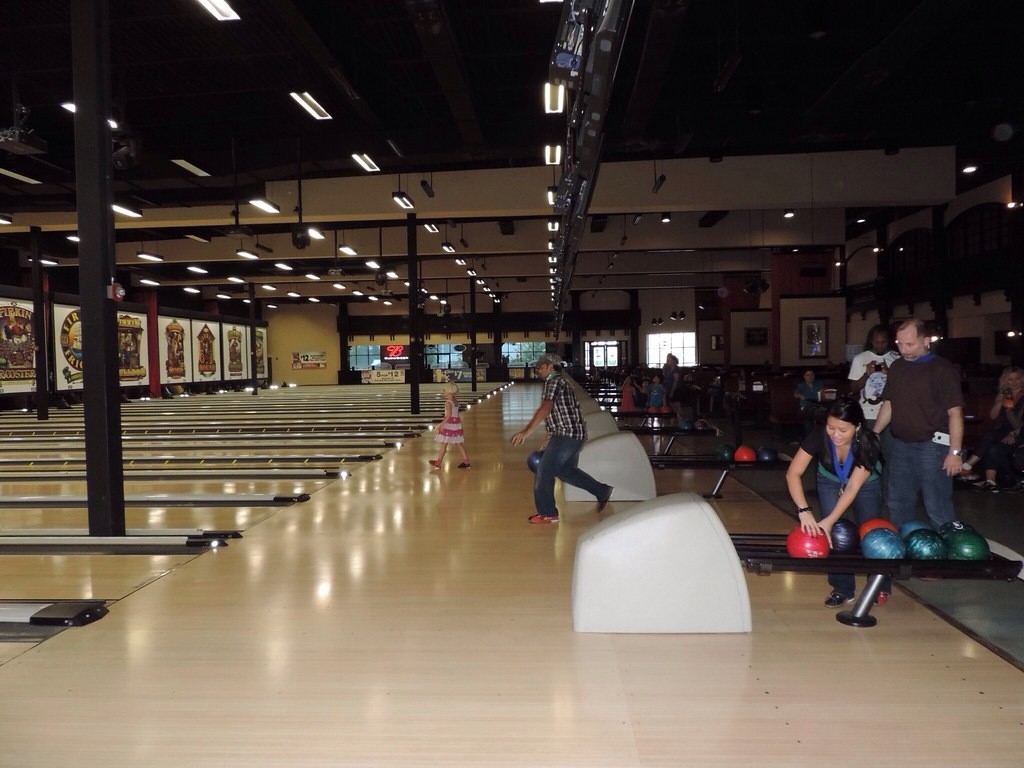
[797,505,813,513]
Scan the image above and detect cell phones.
[932,431,950,447]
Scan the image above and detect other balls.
[715,443,779,462]
[787,518,990,560]
[678,417,710,430]
[527,451,543,473]
[648,405,673,413]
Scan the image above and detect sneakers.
[596,485,613,513]
[876,591,887,604]
[528,513,560,523]
[825,590,855,607]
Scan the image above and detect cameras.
[874,364,882,371]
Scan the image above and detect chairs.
[606,367,843,440]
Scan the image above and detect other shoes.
[972,482,1000,494]
[429,460,442,468]
[1007,482,1024,494]
[957,465,972,476]
[458,462,470,468]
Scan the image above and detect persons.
[621,315,1024,606]
[428,383,470,469]
[511,353,614,523]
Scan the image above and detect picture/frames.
[710,334,723,351]
[745,326,769,346]
[799,317,830,359]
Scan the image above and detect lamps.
[248,191,284,215]
[590,153,686,326]
[542,69,571,117]
[784,207,799,220]
[335,245,362,258]
[0,211,16,227]
[192,0,242,24]
[425,222,442,235]
[351,148,388,176]
[440,240,457,253]
[295,224,325,251]
[184,230,212,243]
[543,182,568,305]
[460,220,470,248]
[544,140,565,167]
[0,162,46,186]
[169,153,214,180]
[285,83,340,126]
[234,246,261,260]
[109,197,145,220]
[420,166,436,198]
[129,260,505,311]
[25,253,64,268]
[390,172,416,210]
[255,231,273,254]
[134,241,166,263]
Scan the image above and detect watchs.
[950,448,963,458]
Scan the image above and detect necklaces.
[834,441,854,497]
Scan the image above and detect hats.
[532,353,561,366]
